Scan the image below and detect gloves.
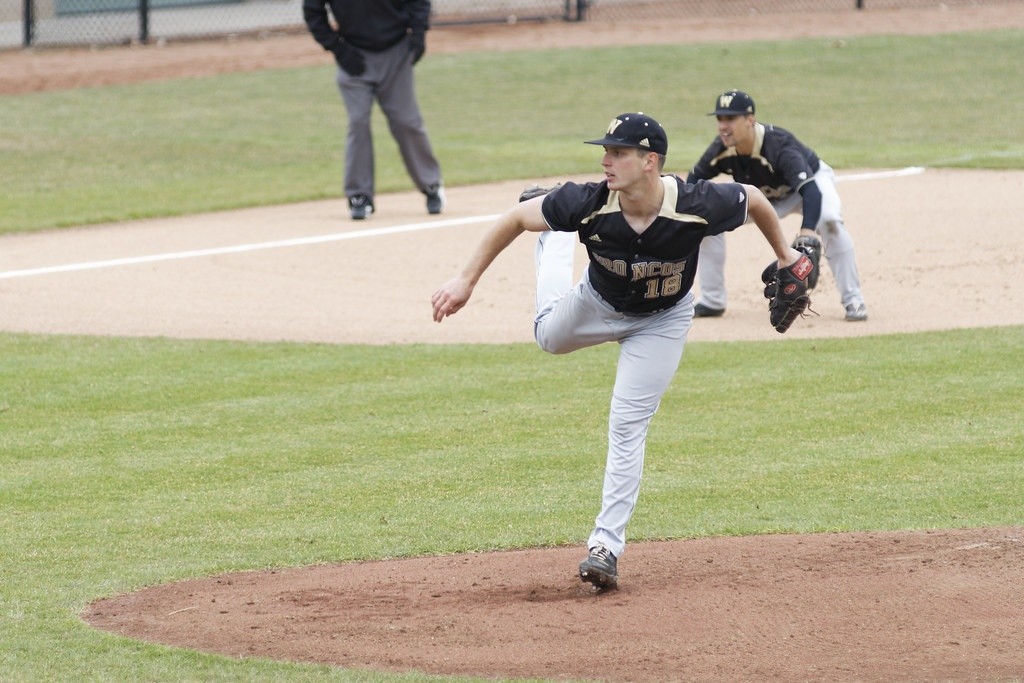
[323,36,368,77]
[406,22,430,65]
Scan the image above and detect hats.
[582,110,669,156]
[705,90,755,119]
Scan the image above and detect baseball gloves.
[761,248,819,334]
[791,234,821,290]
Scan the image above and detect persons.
[684,89,870,322]
[300,0,443,219]
[430,112,816,592]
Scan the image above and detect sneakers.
[348,192,370,219]
[419,186,442,214]
[577,548,621,592]
[843,299,870,321]
[692,303,730,319]
[519,182,569,205]
